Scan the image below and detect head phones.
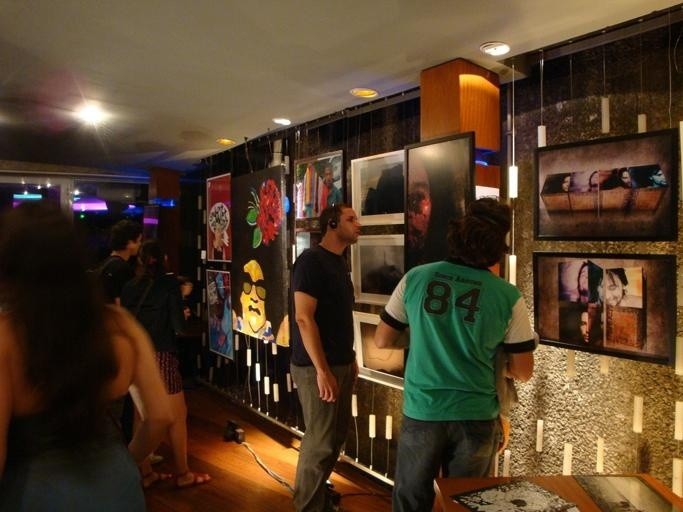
[330,203,338,229]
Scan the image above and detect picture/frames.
[533,127,678,243]
[532,251,676,367]
[206,269,234,361]
[205,173,233,263]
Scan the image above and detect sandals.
[141,470,175,494]
[173,469,212,489]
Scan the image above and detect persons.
[646,163,667,187]
[234,258,270,337]
[605,268,643,309]
[322,162,341,207]
[559,174,569,191]
[1,199,210,512]
[610,168,631,188]
[557,259,604,345]
[288,203,361,512]
[372,196,534,512]
[587,171,598,191]
[408,158,432,255]
